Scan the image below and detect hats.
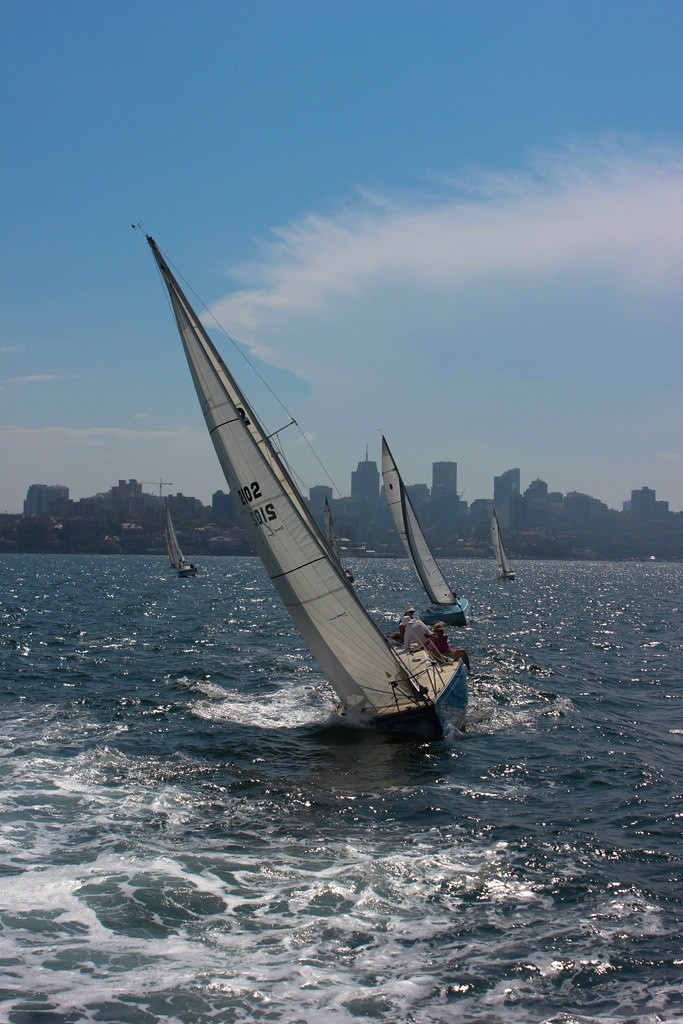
[399,616,416,625]
[406,607,414,612]
[433,623,444,631]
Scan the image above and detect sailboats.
[490,509,516,581]
[379,436,471,626]
[323,497,354,584]
[133,224,467,740]
[163,498,196,579]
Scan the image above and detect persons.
[398,600,414,640]
[399,616,433,652]
[408,623,474,676]
